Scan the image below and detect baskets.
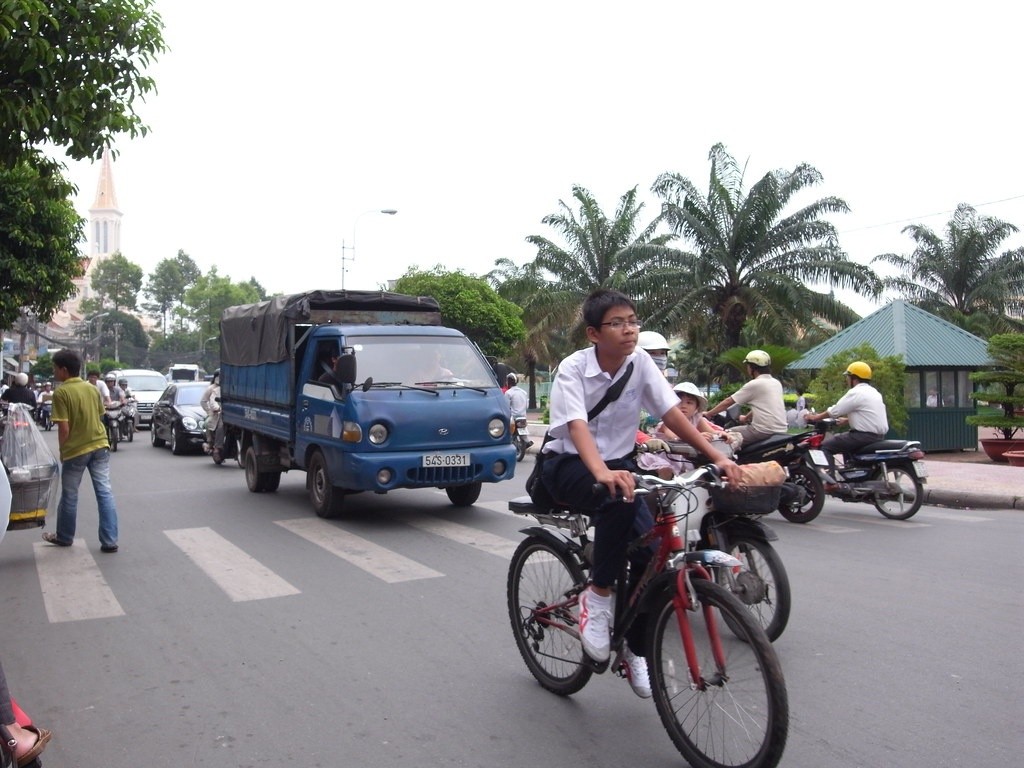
[708,485,782,515]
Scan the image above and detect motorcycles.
[511,416,534,463]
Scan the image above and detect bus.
[165,364,200,385]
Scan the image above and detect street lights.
[202,336,218,355]
[340,208,398,290]
[87,312,109,341]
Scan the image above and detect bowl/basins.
[9,464,56,481]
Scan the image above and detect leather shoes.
[822,483,839,494]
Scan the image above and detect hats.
[674,382,708,413]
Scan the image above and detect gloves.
[644,438,670,454]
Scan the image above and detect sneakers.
[578,589,613,663]
[619,640,652,698]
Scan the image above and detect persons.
[0,664,52,768]
[787,388,809,429]
[318,348,340,383]
[927,387,944,407]
[200,368,220,449]
[529,289,743,698]
[703,350,788,447]
[492,363,529,418]
[42,348,118,553]
[416,350,453,379]
[118,378,139,433]
[0,373,55,427]
[85,370,111,405]
[805,361,889,484]
[636,331,743,448]
[632,382,734,494]
[105,374,128,438]
[209,386,227,464]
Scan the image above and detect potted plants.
[965,333,1024,463]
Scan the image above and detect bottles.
[581,539,594,566]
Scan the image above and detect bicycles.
[504,463,791,768]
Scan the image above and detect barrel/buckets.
[5,477,53,530]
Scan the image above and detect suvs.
[106,368,169,428]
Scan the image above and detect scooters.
[204,397,246,469]
[703,404,828,525]
[801,406,931,521]
[0,387,55,432]
[584,436,791,647]
[103,394,139,453]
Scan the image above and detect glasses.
[122,384,127,386]
[597,320,643,329]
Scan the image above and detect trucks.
[219,289,517,520]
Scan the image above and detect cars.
[149,382,212,456]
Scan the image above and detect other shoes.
[42,532,71,546]
[206,447,214,455]
[101,546,117,552]
[214,453,223,463]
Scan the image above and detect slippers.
[17,724,52,767]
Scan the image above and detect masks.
[650,356,668,372]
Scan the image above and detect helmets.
[636,331,671,357]
[843,361,871,381]
[105,374,116,386]
[742,350,771,367]
[15,373,28,386]
[507,373,518,385]
[119,379,128,388]
